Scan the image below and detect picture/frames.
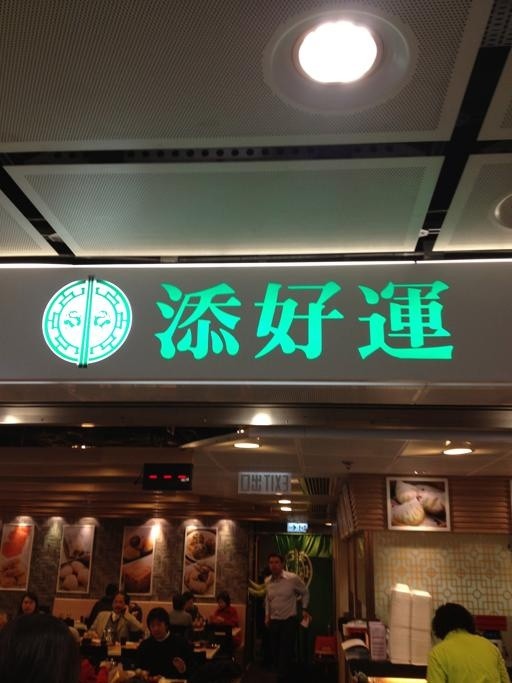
[384,475,453,532]
[0,522,219,598]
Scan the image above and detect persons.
[1,581,239,681]
[262,552,310,681]
[427,601,512,682]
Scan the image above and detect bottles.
[105,628,114,651]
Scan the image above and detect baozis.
[391,480,445,525]
[59,561,89,590]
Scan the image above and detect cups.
[300,617,309,629]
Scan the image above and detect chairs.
[68,611,235,683]
